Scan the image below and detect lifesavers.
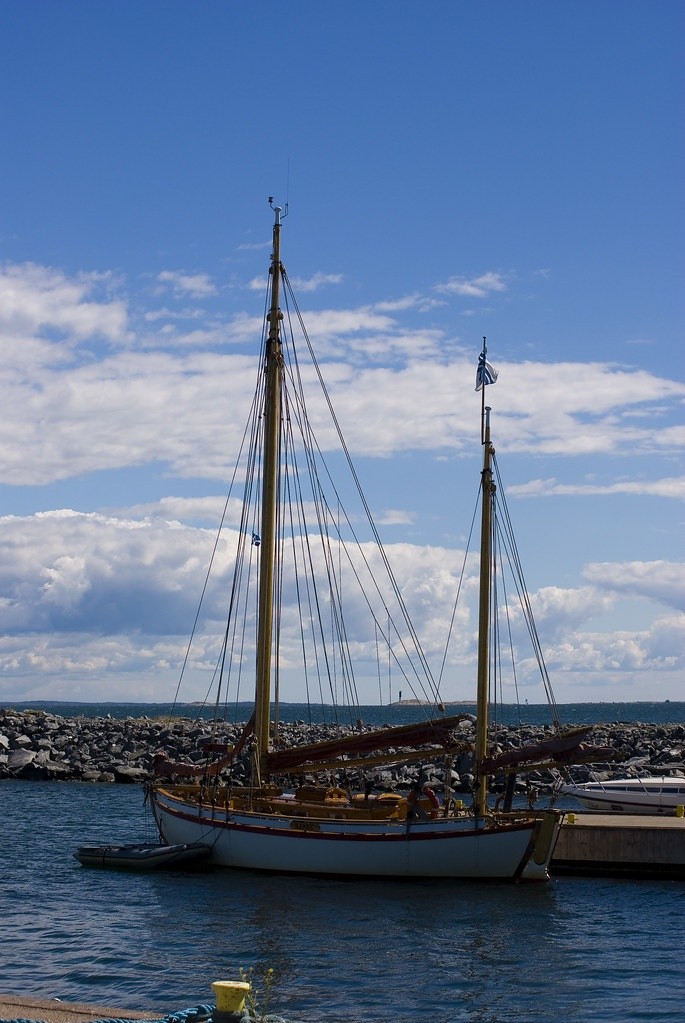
[407,787,439,819]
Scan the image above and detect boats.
[566,771,684,815]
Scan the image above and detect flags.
[474,345,499,392]
[252,533,261,546]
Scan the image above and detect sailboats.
[412,332,684,875]
[73,196,567,886]
